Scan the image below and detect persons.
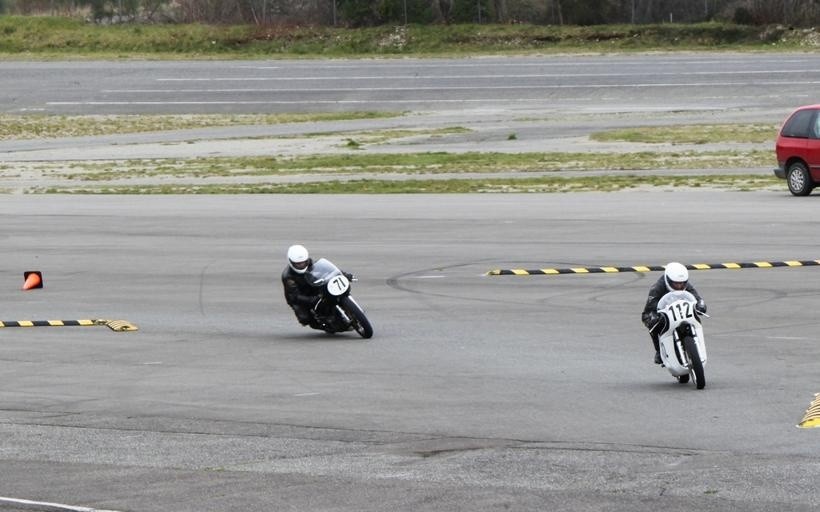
[641,261,707,365]
[281,245,356,329]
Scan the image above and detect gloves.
[346,274,353,282]
[697,303,707,315]
[310,296,320,305]
[647,319,657,329]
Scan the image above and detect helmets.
[664,262,689,292]
[287,245,309,274]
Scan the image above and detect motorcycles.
[304,258,372,339]
[657,290,707,391]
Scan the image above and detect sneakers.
[655,351,663,364]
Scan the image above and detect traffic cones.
[22,271,42,290]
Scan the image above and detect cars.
[773,104,820,195]
